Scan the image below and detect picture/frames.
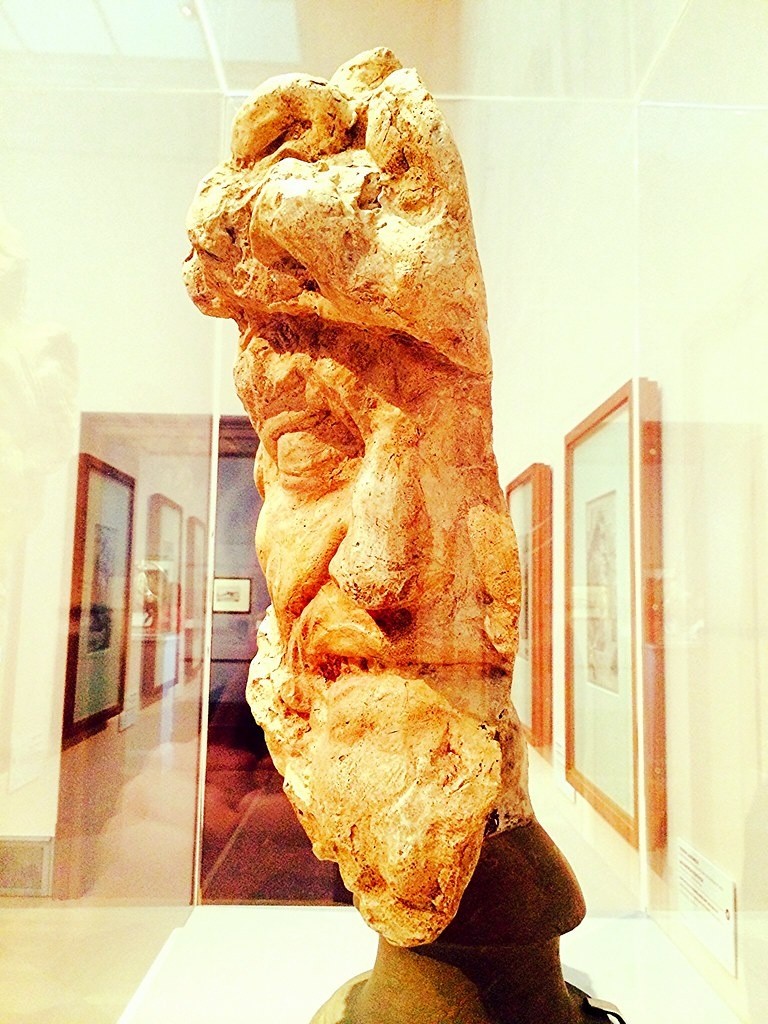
[140,493,184,700]
[62,450,135,742]
[212,576,253,614]
[564,373,668,876]
[184,515,208,679]
[505,462,553,762]
